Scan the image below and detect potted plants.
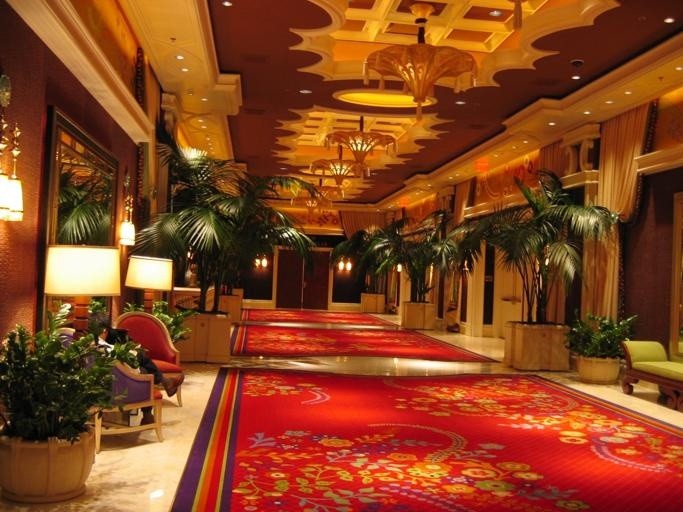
[1,300,118,505]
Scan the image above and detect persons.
[57,312,185,425]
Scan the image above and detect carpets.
[169,370,683,511]
[229,324,503,363]
[240,308,401,327]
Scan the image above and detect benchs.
[621,339,683,414]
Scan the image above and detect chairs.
[49,327,165,454]
[115,310,185,408]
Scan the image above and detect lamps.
[0,173,176,331]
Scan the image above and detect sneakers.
[141,415,154,424]
[166,375,184,396]
